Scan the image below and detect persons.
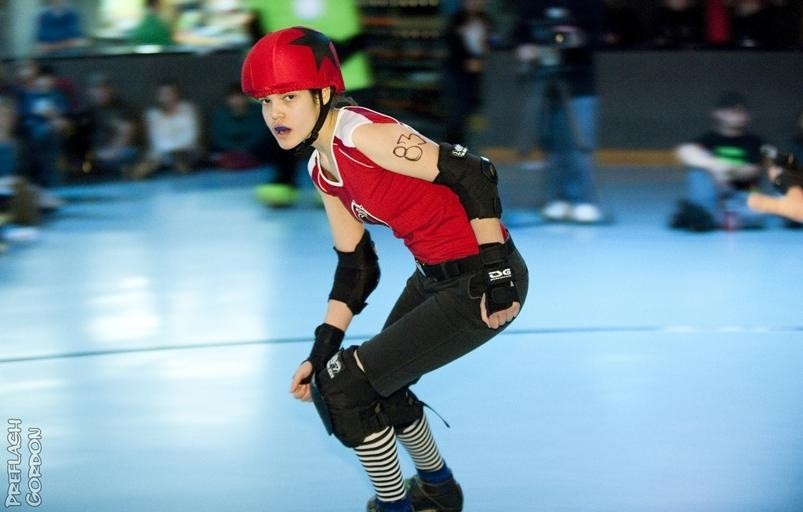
[241,26,528,512]
[1,3,803,255]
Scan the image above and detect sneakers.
[367,474,463,512]
[543,203,596,222]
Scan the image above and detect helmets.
[241,26,344,97]
